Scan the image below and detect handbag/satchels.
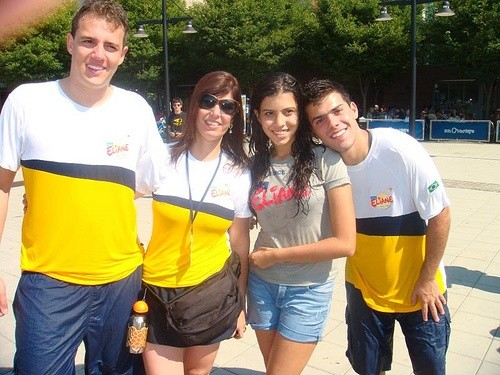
[142,252,243,347]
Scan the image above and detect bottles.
[125,301,148,354]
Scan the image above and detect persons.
[0,0,169,375]
[366,104,465,120]
[167,97,186,142]
[22,71,253,375]
[244,71,356,375]
[300,79,451,375]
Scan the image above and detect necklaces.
[185,148,222,224]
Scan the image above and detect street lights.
[133,0,198,145]
[375,0,456,139]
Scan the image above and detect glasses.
[198,94,242,115]
